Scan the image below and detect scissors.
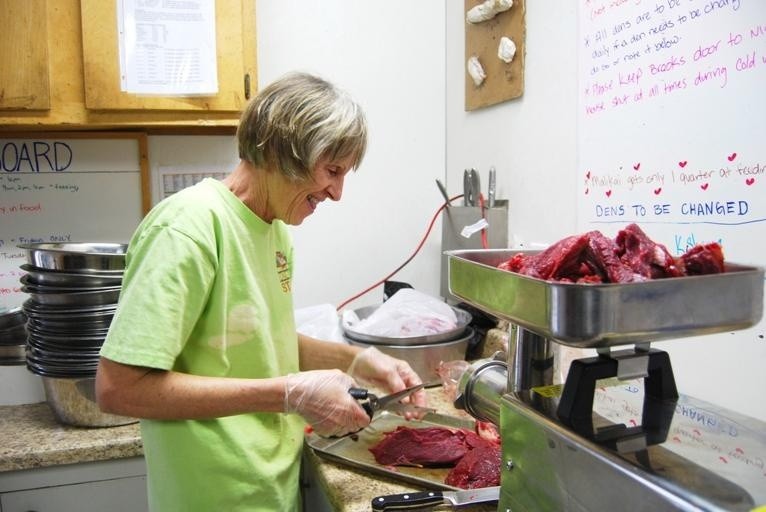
[320,382,430,440]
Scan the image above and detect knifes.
[372,485,501,512]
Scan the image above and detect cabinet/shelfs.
[0,0,258,136]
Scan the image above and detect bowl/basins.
[339,305,471,344]
[343,325,475,387]
[15,242,138,428]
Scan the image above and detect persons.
[92,67,424,512]
